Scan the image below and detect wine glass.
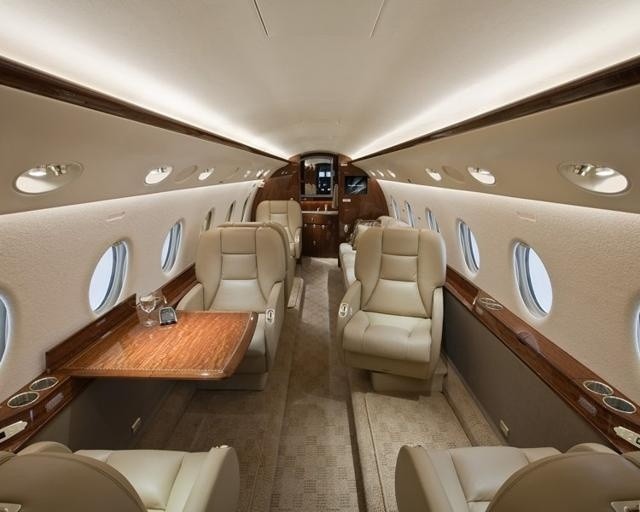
[139,293,159,329]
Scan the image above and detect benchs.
[338,215,412,289]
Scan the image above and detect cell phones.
[158,307,177,325]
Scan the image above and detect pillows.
[349,218,383,250]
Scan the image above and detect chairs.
[304,182,317,195]
[0,434,240,511]
[396,440,639,512]
[176,197,306,394]
[337,223,447,400]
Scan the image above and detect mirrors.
[297,156,333,198]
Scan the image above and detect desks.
[56,310,258,377]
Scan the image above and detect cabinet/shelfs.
[300,213,338,257]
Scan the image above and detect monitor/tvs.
[345,176,367,194]
